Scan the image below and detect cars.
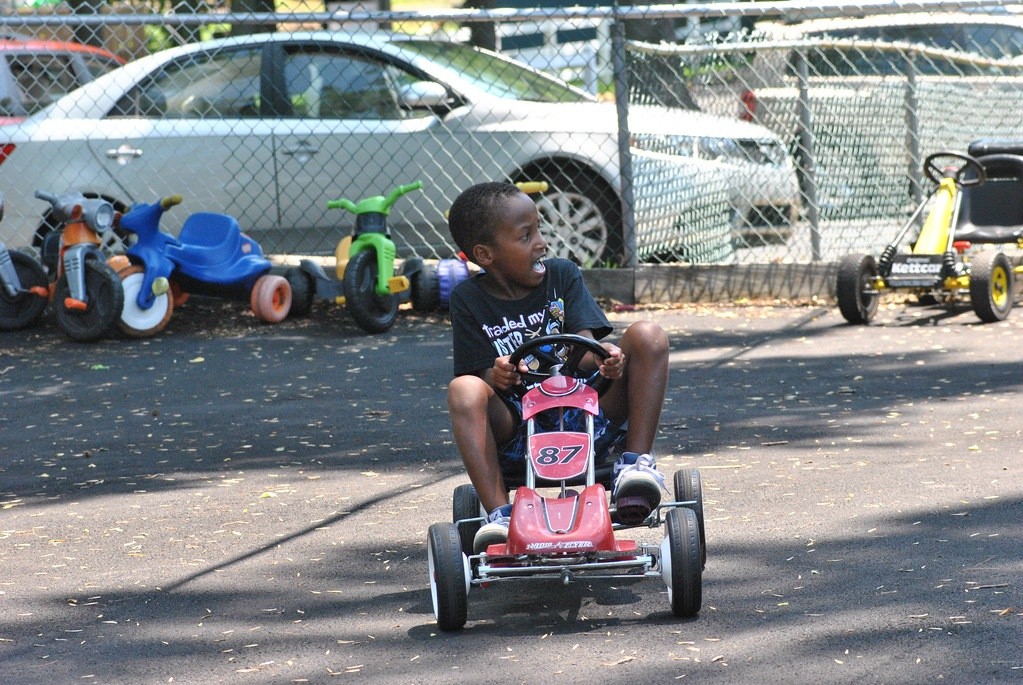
[2,43,130,127]
[0,32,793,272]
[733,5,1023,186]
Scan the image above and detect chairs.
[338,78,381,120]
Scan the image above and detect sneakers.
[471,504,515,555]
[610,452,673,525]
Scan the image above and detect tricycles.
[285,180,440,334]
[1,188,126,347]
[102,194,292,338]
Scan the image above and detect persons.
[449,181,670,554]
